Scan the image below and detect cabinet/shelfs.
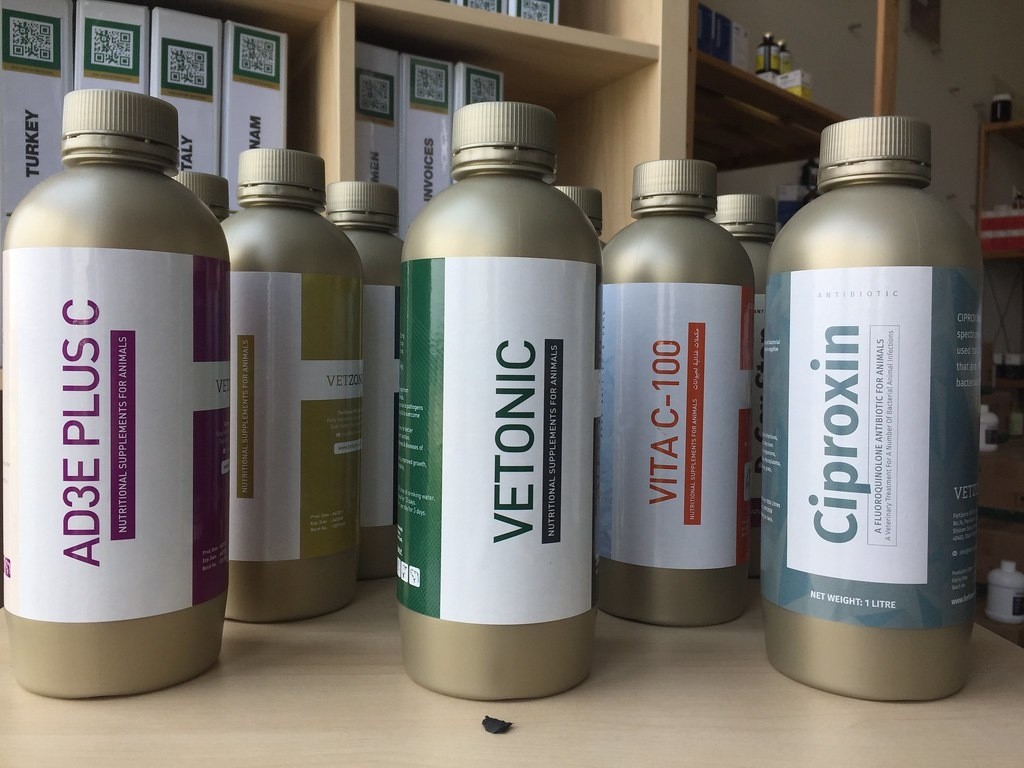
[2,1,1024,768]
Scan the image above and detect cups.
[990,93,1012,124]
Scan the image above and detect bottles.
[398,101,595,702]
[708,193,777,577]
[776,38,792,74]
[756,32,779,86]
[220,148,365,624]
[173,171,229,223]
[326,182,402,583]
[0,89,229,699]
[551,185,607,605]
[597,160,749,627]
[760,116,983,701]
